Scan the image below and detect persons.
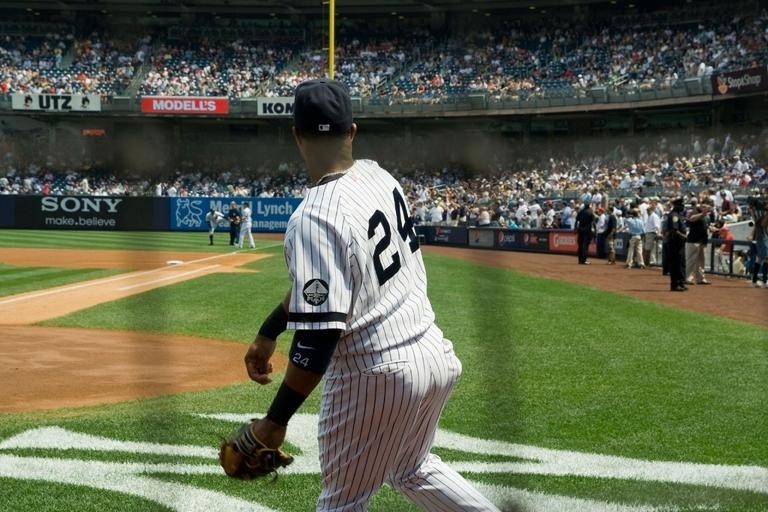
[2,0,767,294]
[212,78,509,512]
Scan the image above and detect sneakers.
[753,280,768,288]
[669,279,712,292]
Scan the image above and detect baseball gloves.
[219,418,293,480]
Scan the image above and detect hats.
[293,77,353,137]
